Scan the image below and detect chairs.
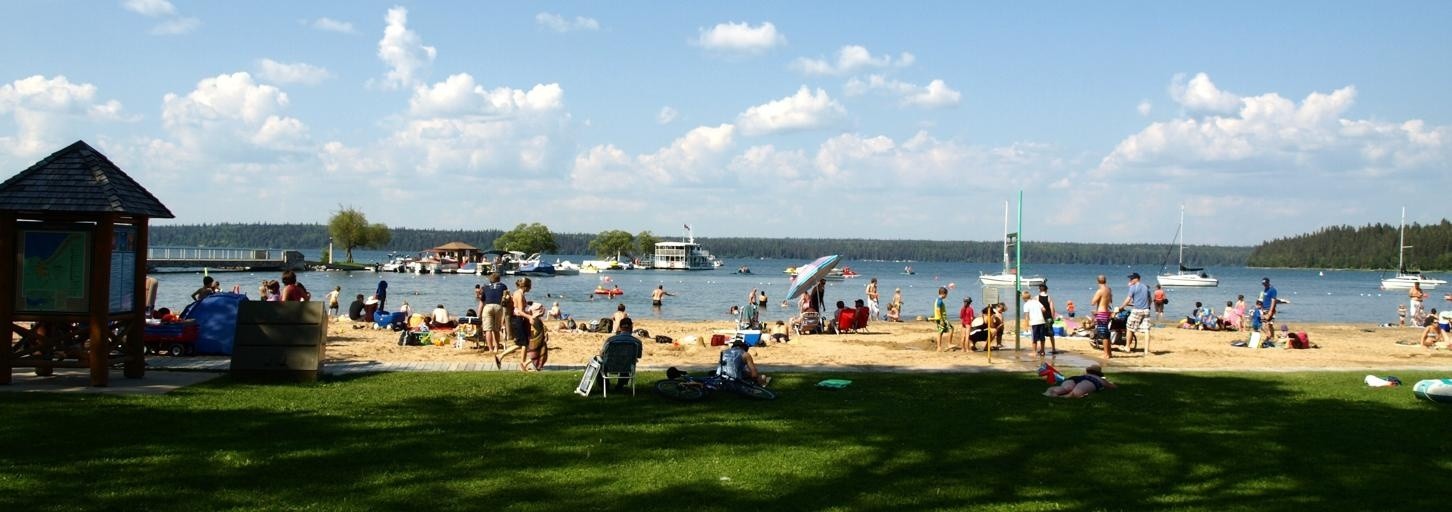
[450,254,885,395]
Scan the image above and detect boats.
[1412,378,1451,399]
[788,268,861,282]
[365,250,582,280]
[582,223,723,275]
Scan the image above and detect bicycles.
[656,351,776,404]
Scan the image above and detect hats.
[1085,364,1104,377]
[1127,272,1140,279]
[620,318,633,328]
[1261,277,1269,284]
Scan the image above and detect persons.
[1396,281,1452,348]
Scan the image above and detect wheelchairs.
[1088,309,1137,353]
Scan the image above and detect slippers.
[1117,345,1131,353]
[495,354,503,370]
[520,363,528,372]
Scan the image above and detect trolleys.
[139,314,200,359]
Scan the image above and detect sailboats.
[979,199,1046,289]
[1155,203,1218,290]
[1379,209,1447,294]
